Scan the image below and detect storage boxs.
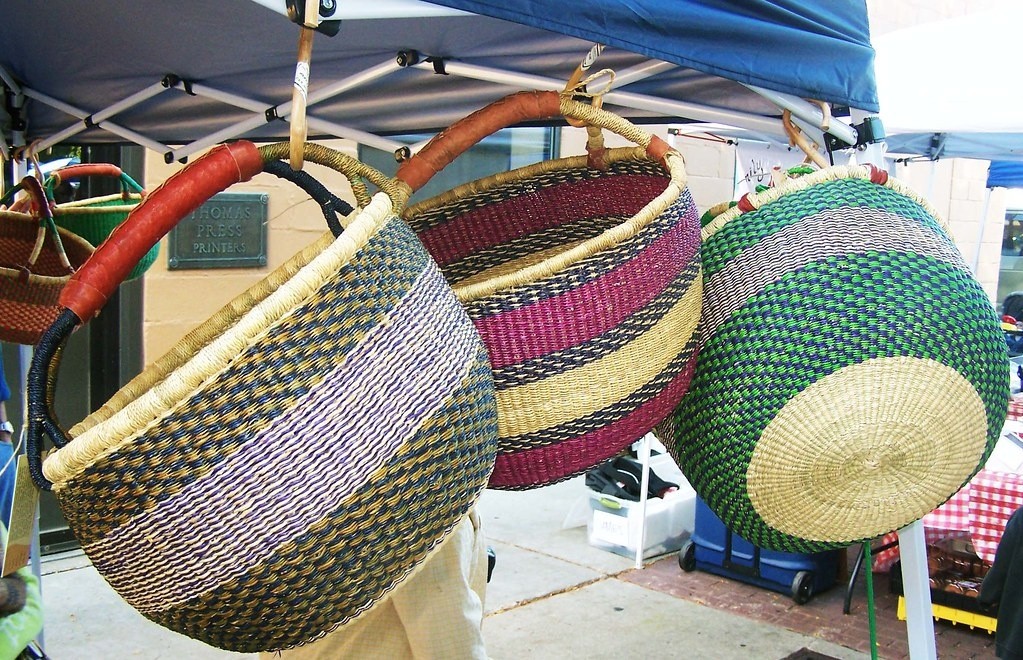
[586,453,698,563]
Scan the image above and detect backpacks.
[584,458,679,501]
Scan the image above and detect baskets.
[384,90,702,491]
[26,141,500,655]
[651,167,1010,554]
[42,163,160,283]
[0,176,99,345]
